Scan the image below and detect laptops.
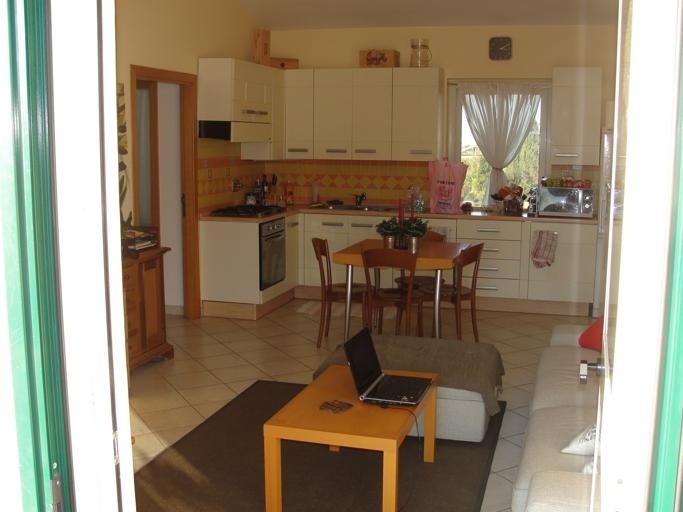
[344,328,432,406]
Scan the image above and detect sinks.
[338,206,386,212]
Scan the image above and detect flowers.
[491,184,524,201]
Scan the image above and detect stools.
[313,335,502,445]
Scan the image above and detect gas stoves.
[209,204,285,217]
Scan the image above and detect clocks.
[489,36,512,60]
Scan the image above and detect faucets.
[356,193,366,208]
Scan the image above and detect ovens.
[260,217,293,291]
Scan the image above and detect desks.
[262,365,436,512]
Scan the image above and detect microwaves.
[537,186,594,218]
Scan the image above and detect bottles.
[262,175,268,193]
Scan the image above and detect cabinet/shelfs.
[294,212,401,303]
[120,245,173,372]
[314,68,351,161]
[457,216,524,313]
[285,69,314,160]
[202,212,296,320]
[393,67,445,162]
[197,57,282,123]
[403,213,457,309]
[551,68,601,167]
[524,217,595,316]
[351,68,391,161]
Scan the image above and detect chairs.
[357,248,423,339]
[419,242,484,345]
[402,231,448,337]
[310,237,374,349]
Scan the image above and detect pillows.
[578,316,605,352]
[562,425,595,454]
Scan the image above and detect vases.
[505,198,521,216]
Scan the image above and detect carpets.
[134,381,508,512]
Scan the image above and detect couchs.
[512,323,602,512]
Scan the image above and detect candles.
[410,192,416,219]
[398,198,404,226]
